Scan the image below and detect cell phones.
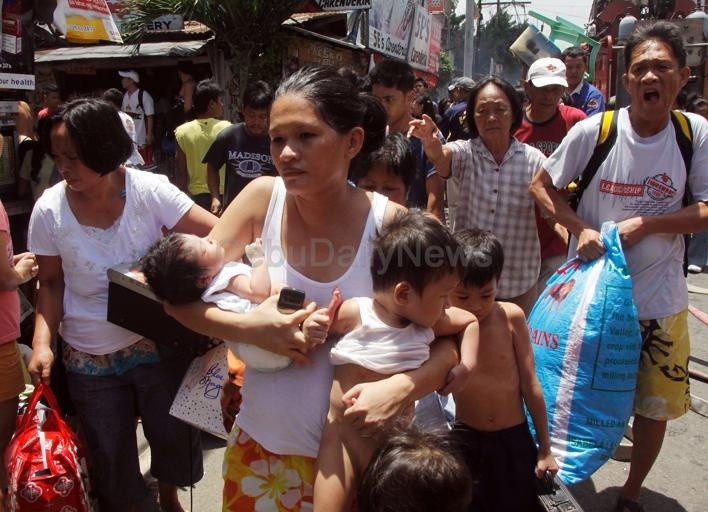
[277,286,307,310]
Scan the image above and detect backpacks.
[567,108,693,267]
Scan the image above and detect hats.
[447,76,476,92]
[527,57,569,88]
[118,70,139,83]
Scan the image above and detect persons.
[0,19,708,512]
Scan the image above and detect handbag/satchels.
[166,342,235,442]
[0,396,97,512]
